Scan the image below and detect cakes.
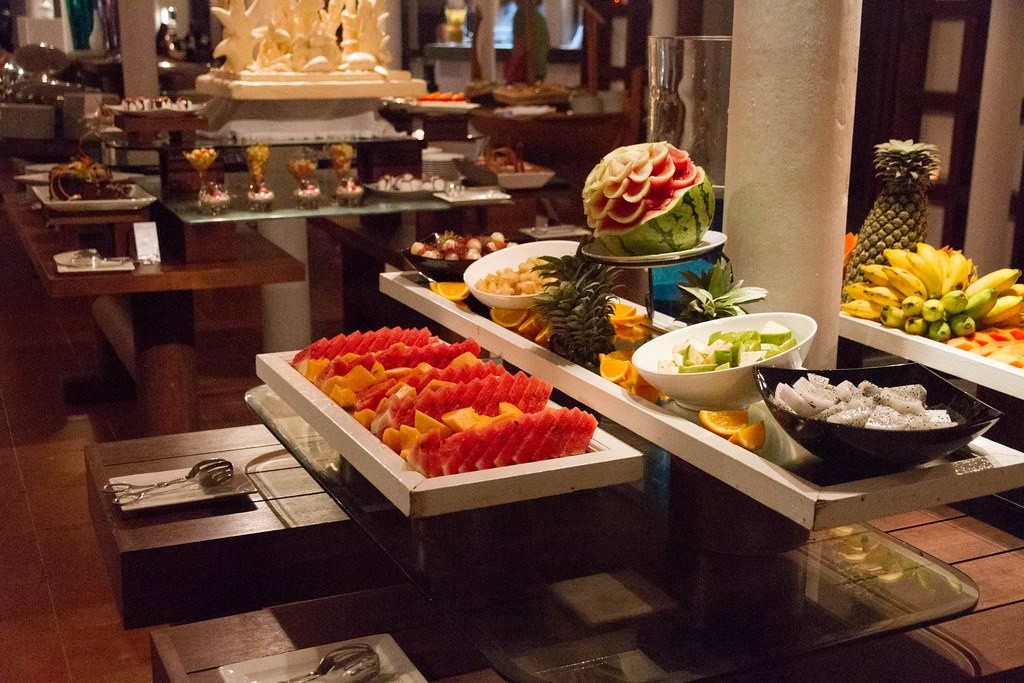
[49,153,133,201]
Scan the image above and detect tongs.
[101,458,234,506]
[278,642,379,683]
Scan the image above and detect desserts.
[199,179,365,215]
[379,173,445,192]
[121,97,194,111]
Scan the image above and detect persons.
[509,0,552,82]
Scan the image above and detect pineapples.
[842,139,942,303]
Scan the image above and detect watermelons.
[291,326,598,479]
[582,141,716,257]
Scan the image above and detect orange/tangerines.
[429,282,471,301]
[490,308,552,346]
[598,350,669,402]
[606,302,646,327]
[699,409,767,452]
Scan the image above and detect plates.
[581,229,727,264]
[104,101,207,117]
[362,181,446,200]
[433,189,512,204]
[31,181,157,214]
[109,465,257,511]
[401,231,479,282]
[218,632,428,683]
[631,309,820,414]
[753,361,1005,471]
[12,173,50,185]
[462,239,581,308]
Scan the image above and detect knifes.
[56,256,136,273]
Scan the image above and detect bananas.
[840,243,1024,340]
